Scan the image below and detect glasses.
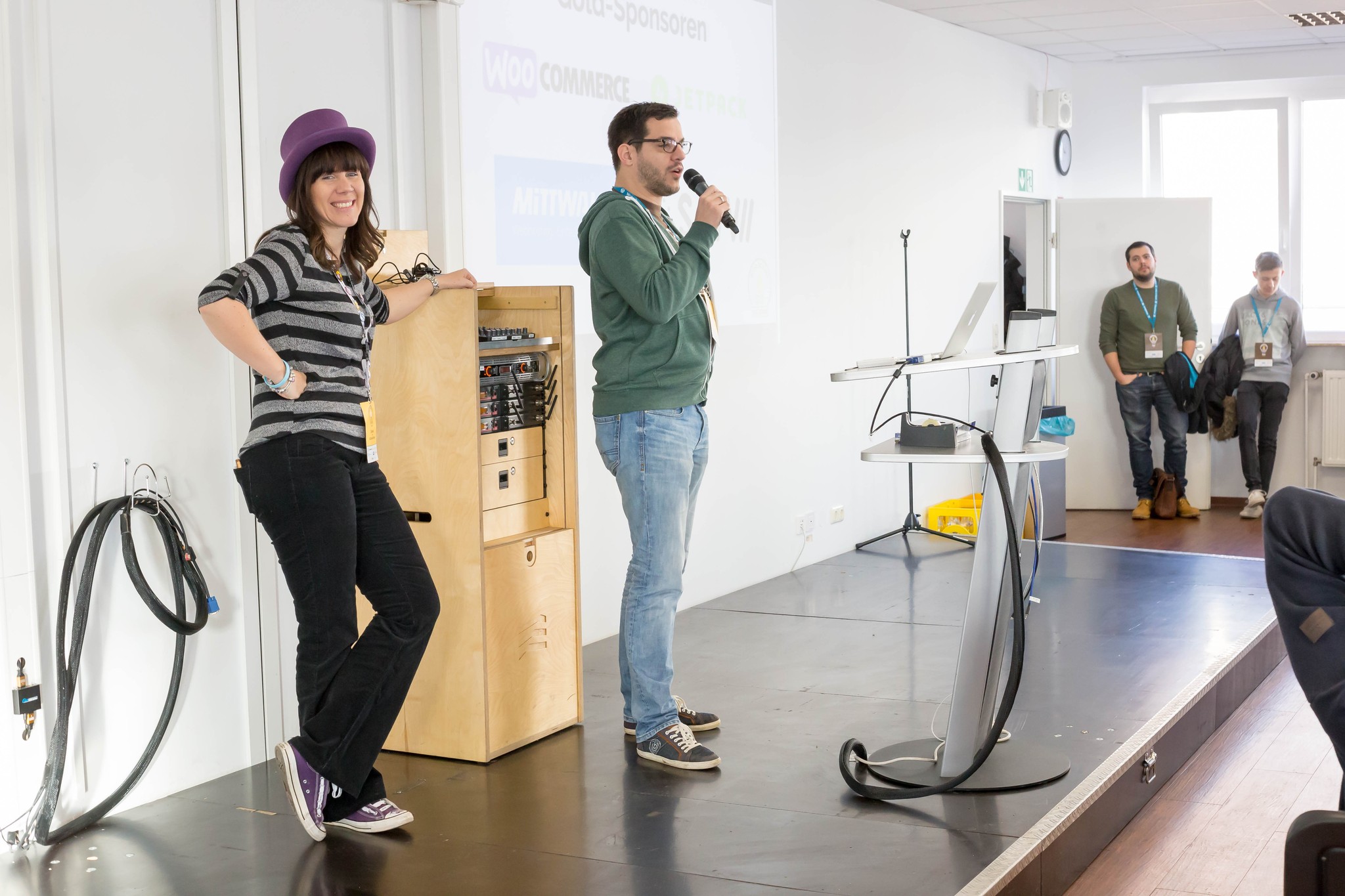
[627,139,692,154]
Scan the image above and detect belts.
[1128,372,1162,377]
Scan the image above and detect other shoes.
[1132,499,1155,519]
[1177,498,1201,518]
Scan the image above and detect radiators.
[1321,370,1345,467]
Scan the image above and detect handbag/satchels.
[1149,468,1177,519]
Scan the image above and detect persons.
[199,108,476,841]
[1098,241,1200,519]
[1264,485,1345,811]
[1218,251,1306,518]
[579,103,730,769]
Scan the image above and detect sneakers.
[275,742,342,842]
[322,798,414,833]
[624,695,721,735]
[1240,504,1263,518]
[637,722,721,769]
[1248,489,1266,508]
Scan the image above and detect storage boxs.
[927,493,1024,539]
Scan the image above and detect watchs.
[424,274,440,296]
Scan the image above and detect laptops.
[895,281,997,363]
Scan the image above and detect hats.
[278,108,376,213]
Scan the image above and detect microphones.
[682,169,739,234]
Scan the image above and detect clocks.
[1056,130,1072,175]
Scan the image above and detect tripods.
[855,229,976,550]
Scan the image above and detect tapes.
[922,419,941,427]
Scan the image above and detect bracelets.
[263,359,295,394]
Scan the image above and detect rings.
[717,196,724,204]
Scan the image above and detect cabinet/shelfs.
[830,309,1072,794]
[351,230,585,764]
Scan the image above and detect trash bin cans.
[1040,405,1066,541]
